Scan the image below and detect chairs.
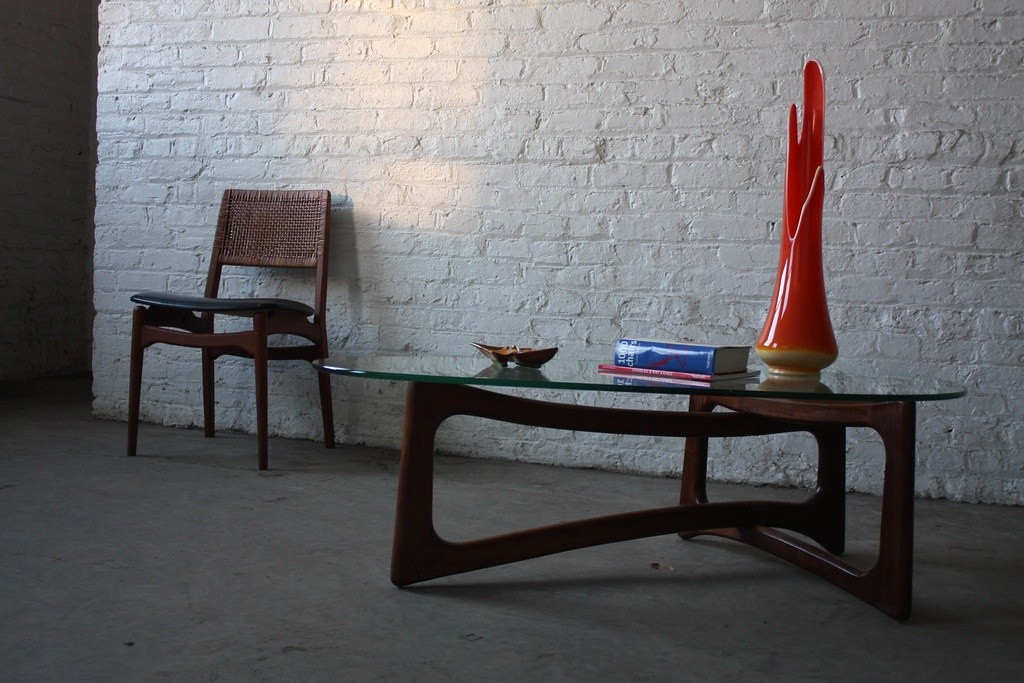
[125,187,336,472]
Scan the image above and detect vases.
[756,58,838,378]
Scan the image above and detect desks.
[311,355,967,624]
